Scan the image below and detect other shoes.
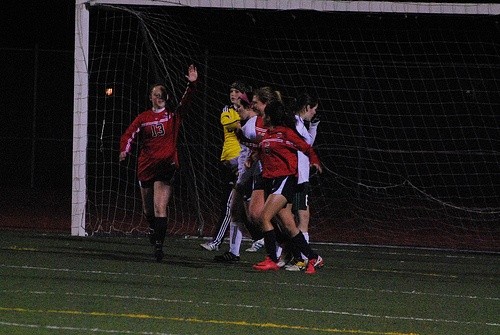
[151,241,164,261]
[285,260,306,272]
[304,255,323,267]
[284,257,302,267]
[276,251,294,267]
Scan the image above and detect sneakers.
[304,257,317,274]
[200,242,219,252]
[251,257,280,271]
[245,237,265,252]
[214,251,240,264]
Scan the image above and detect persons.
[118,65,198,260]
[200,81,326,275]
